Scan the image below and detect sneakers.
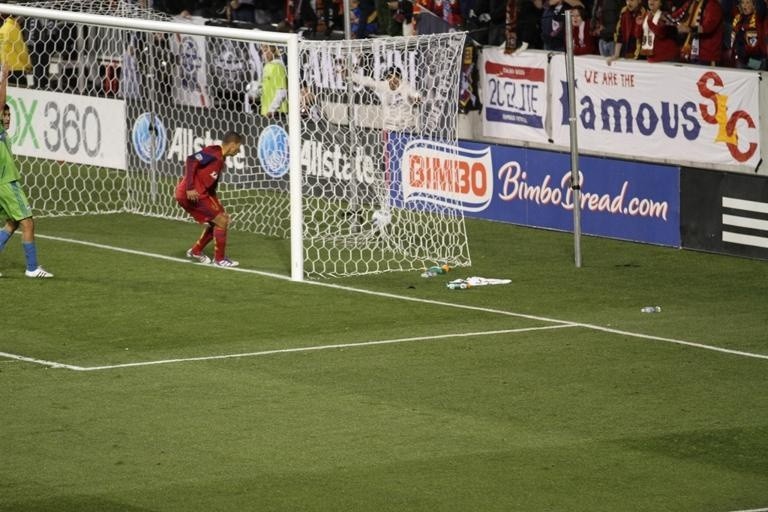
[25,264,53,278]
[212,257,240,267]
[186,247,211,264]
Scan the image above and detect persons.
[173,130,241,268]
[1,0,348,122]
[345,0,768,132]
[0,62,56,281]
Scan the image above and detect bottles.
[640,305,662,313]
